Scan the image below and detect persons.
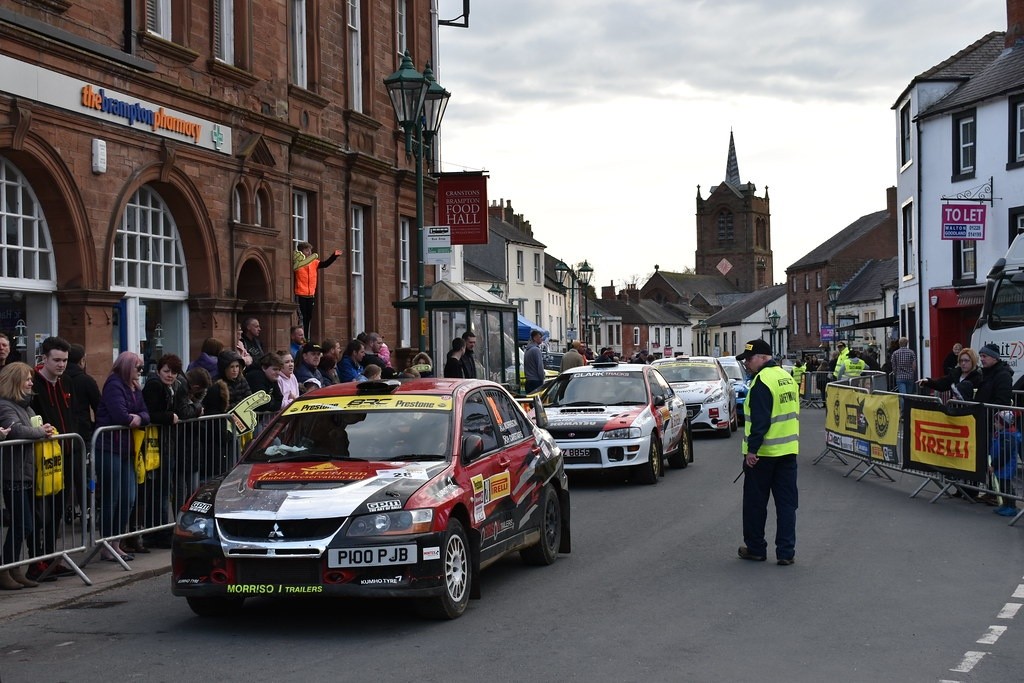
[791,359,805,398]
[833,342,850,379]
[988,410,1021,517]
[915,348,983,497]
[816,352,838,408]
[799,359,807,372]
[837,350,870,387]
[806,354,820,372]
[891,337,916,419]
[943,343,963,408]
[444,331,477,379]
[0,317,438,590]
[962,343,1014,499]
[951,380,987,499]
[856,346,898,392]
[292,242,343,341]
[735,339,800,565]
[521,331,660,396]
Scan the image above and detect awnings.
[836,315,899,333]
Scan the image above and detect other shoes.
[101,550,119,562]
[9,567,40,588]
[777,556,794,565]
[120,535,150,553]
[986,498,998,507]
[962,492,978,499]
[952,491,963,497]
[999,507,1017,516]
[992,505,1008,512]
[0,570,25,590]
[738,546,767,560]
[975,493,996,502]
[115,547,135,560]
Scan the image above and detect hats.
[303,342,326,353]
[994,409,1021,428]
[736,339,771,360]
[838,342,845,347]
[979,344,1000,360]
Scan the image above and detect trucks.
[971,228,1023,466]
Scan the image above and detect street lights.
[556,259,595,358]
[826,281,841,352]
[769,310,781,357]
[699,320,707,356]
[383,48,453,367]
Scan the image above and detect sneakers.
[26,563,58,582]
[49,562,74,576]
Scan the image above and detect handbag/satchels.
[34,425,64,496]
[132,428,145,484]
[145,425,160,472]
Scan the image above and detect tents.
[516,313,551,368]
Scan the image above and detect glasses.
[136,366,143,372]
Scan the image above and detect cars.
[717,357,751,422]
[524,363,694,484]
[648,355,738,438]
[169,379,572,621]
